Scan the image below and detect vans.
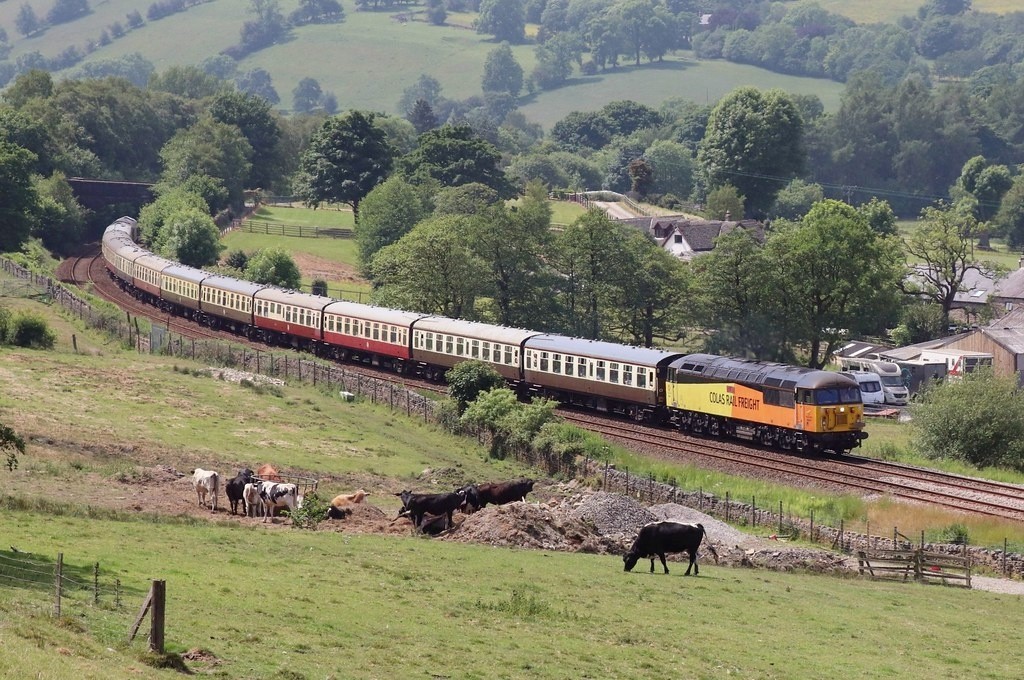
[838,369,884,404]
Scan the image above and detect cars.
[822,326,849,336]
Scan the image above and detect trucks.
[841,358,910,405]
[919,349,993,386]
[899,359,947,395]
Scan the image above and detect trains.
[102,216,868,460]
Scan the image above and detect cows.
[622,520,719,575]
[190,468,219,513]
[394,477,534,536]
[324,490,370,520]
[226,465,300,526]
